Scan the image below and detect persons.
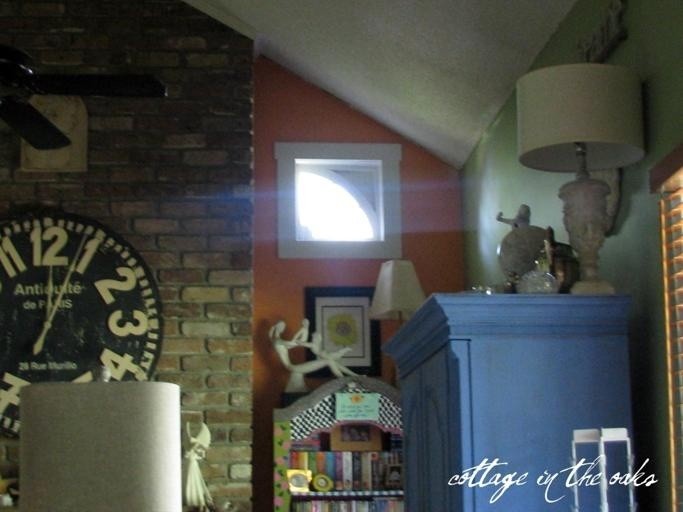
[293,332,354,362]
[495,202,530,230]
[291,318,310,346]
[269,320,361,385]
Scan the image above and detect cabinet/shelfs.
[382,291,635,511]
[271,374,404,511]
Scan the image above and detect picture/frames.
[329,424,382,451]
[304,287,381,378]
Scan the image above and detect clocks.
[1,208,164,439]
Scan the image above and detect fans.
[0,38,166,148]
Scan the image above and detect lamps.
[517,63,646,295]
[368,260,426,327]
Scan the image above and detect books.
[288,448,407,512]
